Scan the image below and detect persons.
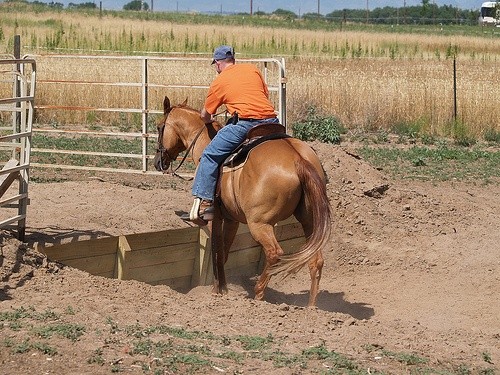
[180,45,280,221]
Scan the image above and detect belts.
[239,118,264,122]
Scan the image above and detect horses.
[154,96,334,307]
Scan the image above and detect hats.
[211,46,235,65]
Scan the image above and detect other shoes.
[180,201,214,220]
